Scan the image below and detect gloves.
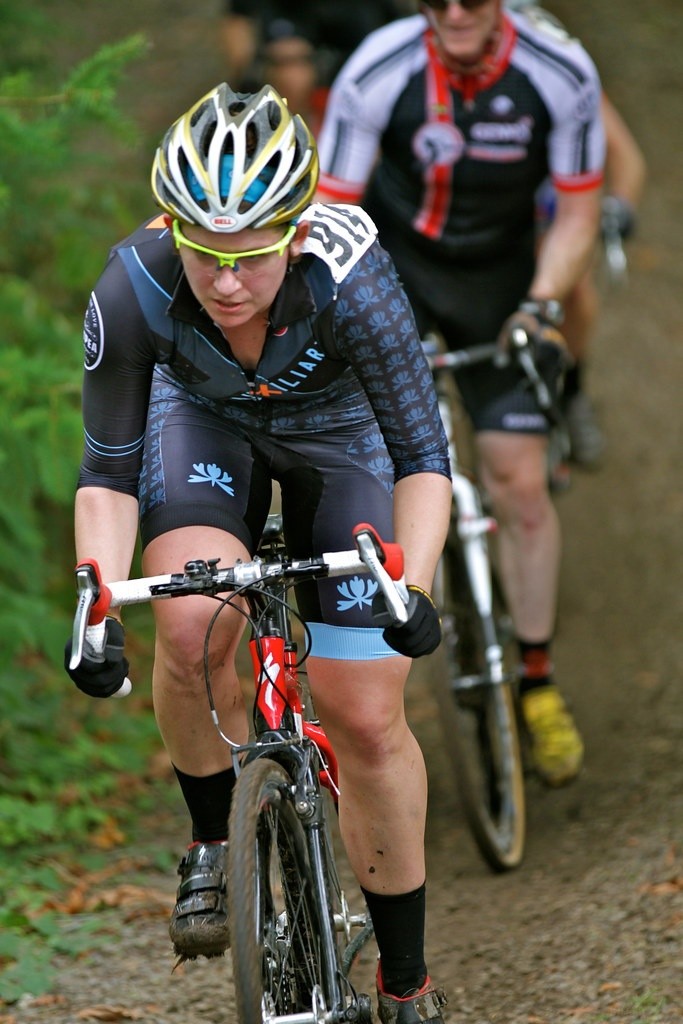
[62,616,131,700]
[496,309,577,383]
[371,585,443,659]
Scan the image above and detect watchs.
[519,300,565,325]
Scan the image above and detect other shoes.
[566,396,609,477]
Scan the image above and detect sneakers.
[522,686,584,789]
[374,951,449,1024]
[168,840,230,954]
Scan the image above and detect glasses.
[171,212,300,279]
[423,0,490,13]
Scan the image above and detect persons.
[537,84,649,471]
[219,2,399,113]
[67,84,475,1023]
[315,1,608,787]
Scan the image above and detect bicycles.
[415,322,574,874]
[530,181,631,493]
[70,510,450,1022]
[219,48,341,155]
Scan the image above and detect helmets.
[149,80,320,234]
